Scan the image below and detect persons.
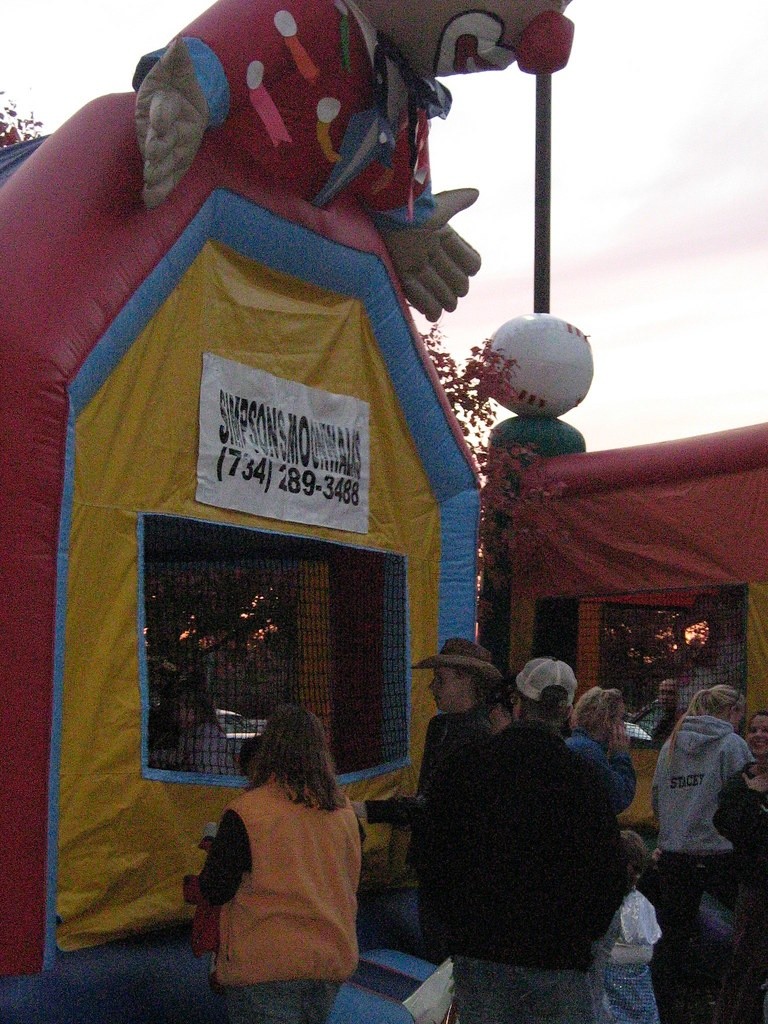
[652,679,676,738]
[565,687,636,815]
[650,684,756,1008]
[199,704,367,1024]
[348,639,502,962]
[589,830,662,1024]
[449,656,629,1024]
[713,710,768,1024]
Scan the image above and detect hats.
[515,657,578,707]
[411,638,502,682]
